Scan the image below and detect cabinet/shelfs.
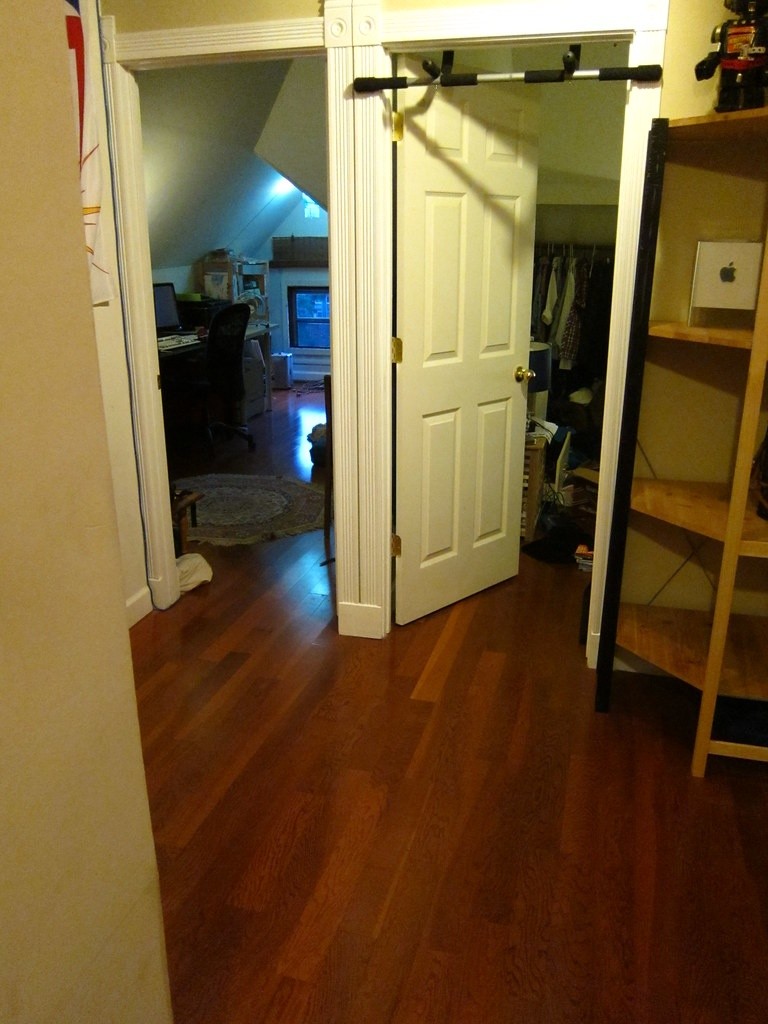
[588,106,768,778]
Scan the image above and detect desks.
[521,437,548,543]
[157,323,278,413]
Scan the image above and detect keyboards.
[157,334,196,349]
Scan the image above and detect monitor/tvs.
[153,283,183,331]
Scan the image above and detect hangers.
[533,241,602,277]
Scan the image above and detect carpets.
[177,472,333,548]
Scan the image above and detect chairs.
[164,302,256,460]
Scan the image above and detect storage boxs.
[175,298,232,327]
[269,352,294,389]
